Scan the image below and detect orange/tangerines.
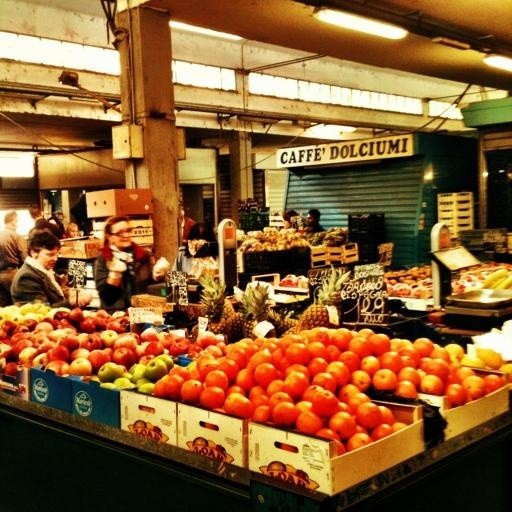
[383,260,512,296]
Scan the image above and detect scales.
[427,246,512,332]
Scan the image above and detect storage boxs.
[247,399,428,496]
[417,365,512,443]
[0,363,121,430]
[119,388,178,448]
[176,401,251,469]
[309,242,393,268]
[53,187,154,260]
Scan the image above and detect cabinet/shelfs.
[436,190,475,244]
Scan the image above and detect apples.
[0,308,512,458]
[238,227,310,253]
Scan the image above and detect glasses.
[107,227,132,236]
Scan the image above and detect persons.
[26,205,48,239]
[0,210,27,307]
[94,216,153,316]
[9,231,93,309]
[46,210,65,236]
[57,222,85,239]
[303,209,326,235]
[280,209,301,228]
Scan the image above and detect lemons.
[0,301,49,321]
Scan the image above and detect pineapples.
[192,264,352,336]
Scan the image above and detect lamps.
[423,162,435,180]
[311,5,410,41]
[482,51,512,74]
